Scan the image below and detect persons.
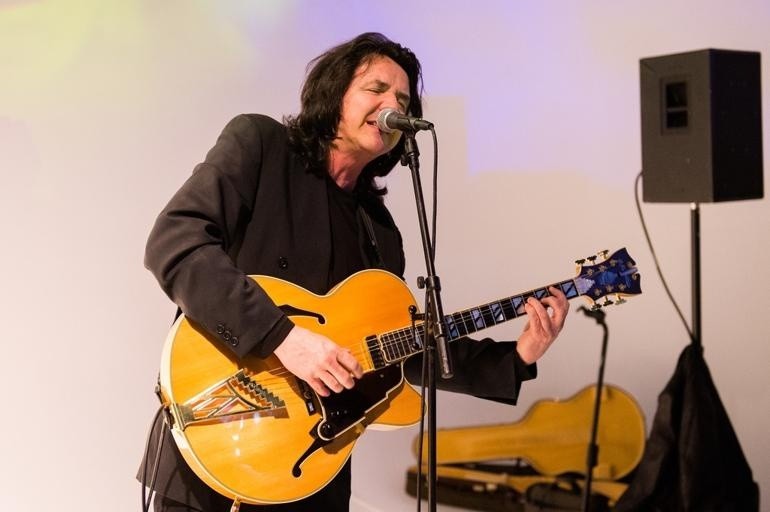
[136,31,568,512]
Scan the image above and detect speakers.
[640,49,764,202]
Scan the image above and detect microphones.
[377,108,434,133]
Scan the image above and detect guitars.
[153,248,642,505]
